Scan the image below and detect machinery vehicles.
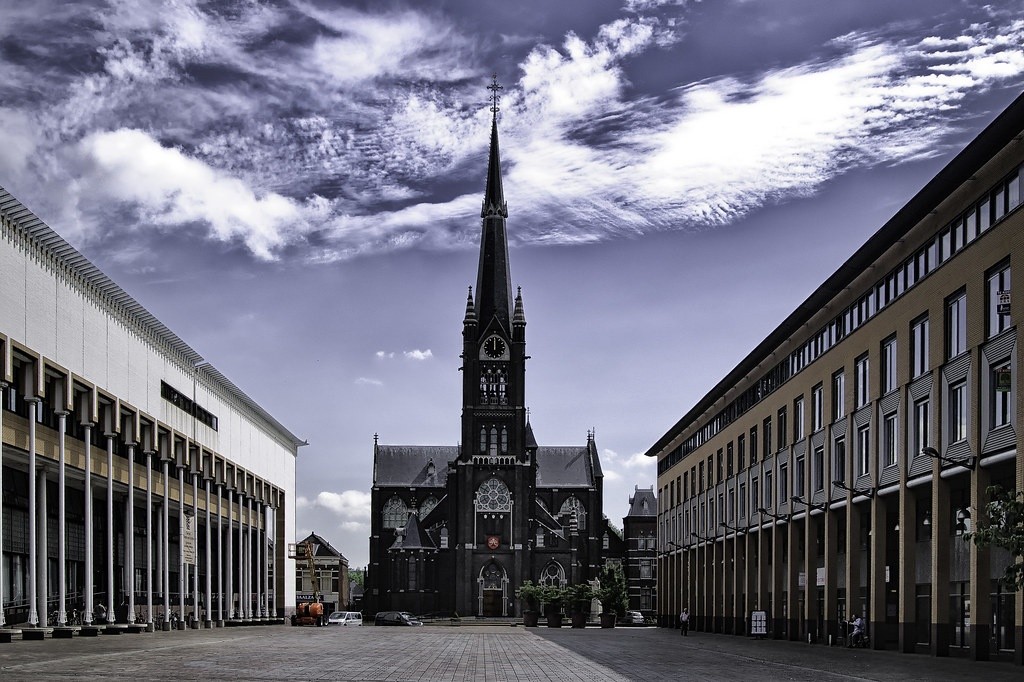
[287,541,329,627]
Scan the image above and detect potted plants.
[514,579,543,627]
[567,583,593,628]
[541,585,567,628]
[593,587,621,629]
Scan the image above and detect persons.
[847,613,866,648]
[94,600,106,625]
[680,608,691,637]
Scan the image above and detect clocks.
[480,334,510,360]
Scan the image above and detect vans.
[330,611,363,627]
[376,611,423,626]
[617,611,645,623]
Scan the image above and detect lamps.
[647,548,670,556]
[789,496,828,513]
[921,447,977,471]
[758,507,790,523]
[668,541,689,551]
[719,522,748,534]
[690,532,715,543]
[832,480,875,499]
